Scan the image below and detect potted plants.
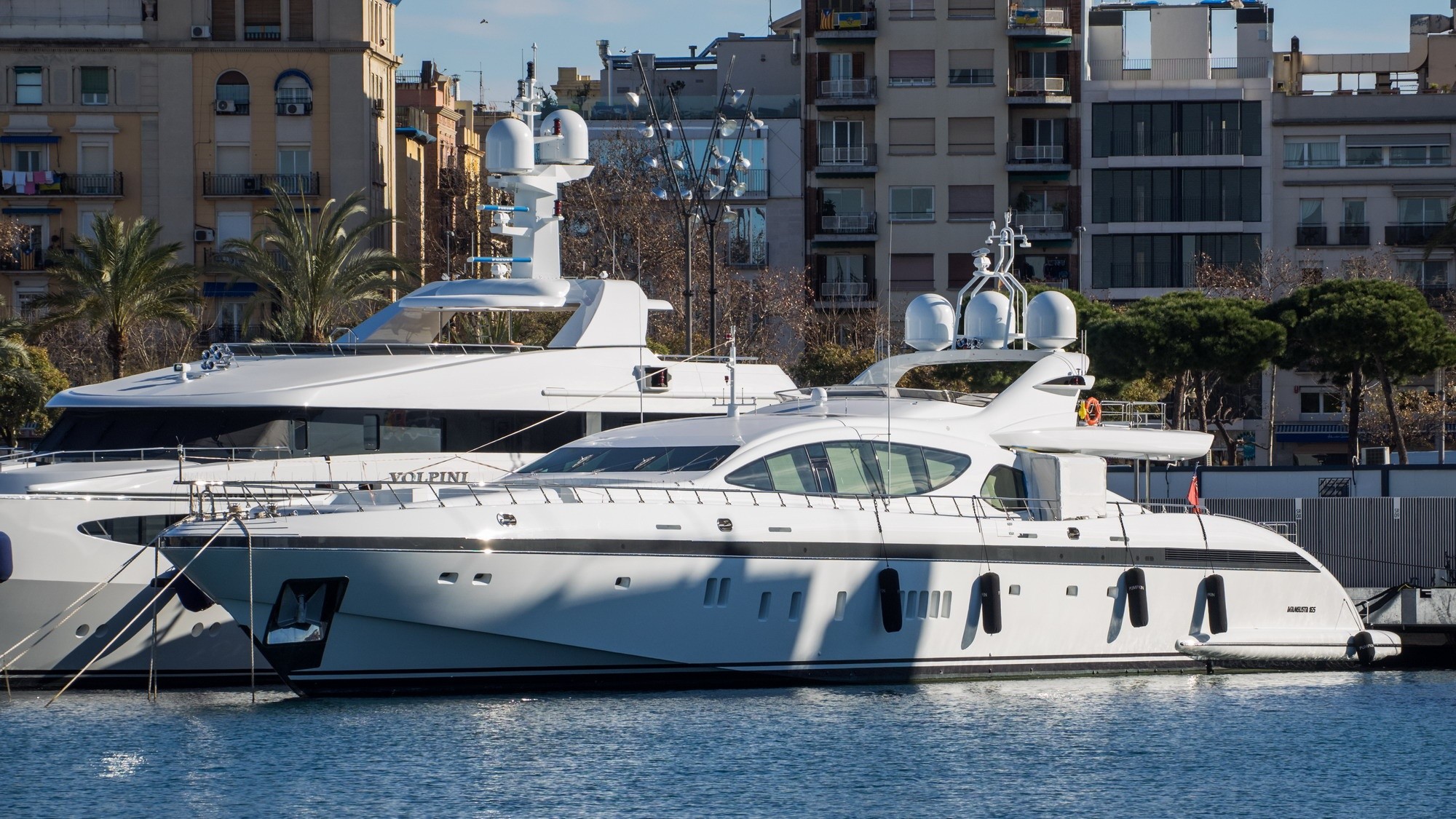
[1421,83,1439,94]
[1441,83,1451,94]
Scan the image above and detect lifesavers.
[1085,397,1101,426]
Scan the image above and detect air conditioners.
[191,25,210,38]
[243,178,262,191]
[376,99,385,111]
[193,229,214,242]
[216,99,236,112]
[286,104,305,115]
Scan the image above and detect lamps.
[624,79,764,224]
[201,342,235,370]
[173,362,193,384]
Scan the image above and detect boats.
[154,203,1370,705]
[0,78,821,689]
